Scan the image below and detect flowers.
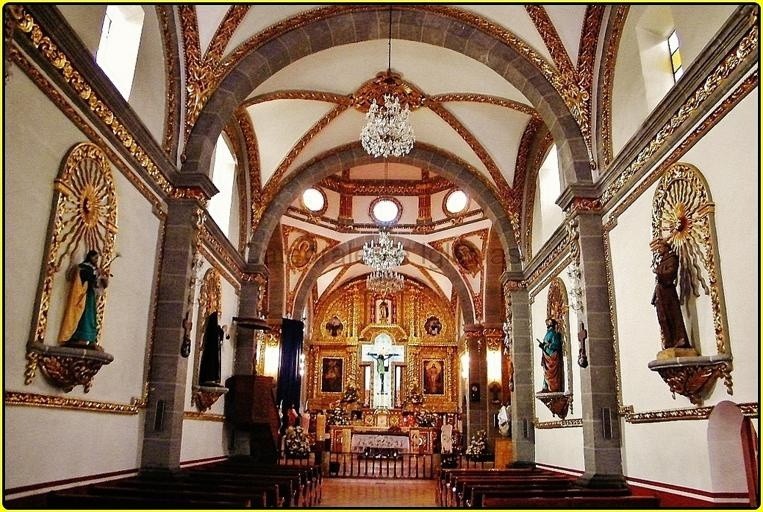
[416,409,437,427]
[327,407,351,425]
[286,427,312,457]
[465,429,489,458]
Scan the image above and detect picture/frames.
[421,311,447,341]
[320,356,346,394]
[319,307,347,341]
[422,359,445,397]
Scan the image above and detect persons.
[286,403,299,427]
[537,319,562,392]
[324,360,339,383]
[650,242,692,348]
[298,408,311,435]
[198,311,226,385]
[427,363,438,385]
[372,355,391,382]
[54,249,110,346]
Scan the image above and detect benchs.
[47,463,323,508]
[434,467,661,508]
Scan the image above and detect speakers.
[602,406,613,439]
[152,398,165,430]
[523,419,530,438]
[494,414,499,427]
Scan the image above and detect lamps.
[360,4,417,161]
[359,224,406,303]
